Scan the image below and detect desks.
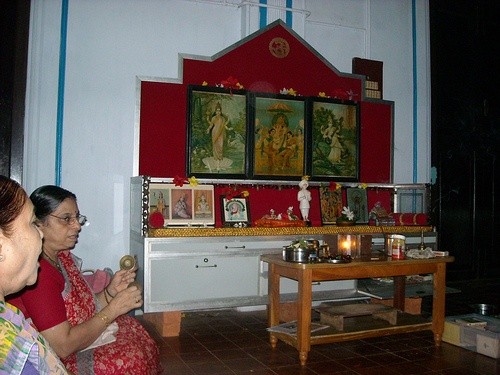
[261,252,455,366]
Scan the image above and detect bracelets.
[103,288,110,304]
[106,288,114,298]
[103,306,118,319]
[93,312,110,327]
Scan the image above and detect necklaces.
[42,250,63,273]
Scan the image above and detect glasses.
[45,211,86,227]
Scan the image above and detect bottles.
[390,235,406,259]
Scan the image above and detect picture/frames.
[219,195,251,227]
[148,182,216,226]
[308,95,361,182]
[250,92,309,181]
[346,187,370,225]
[318,187,344,226]
[185,84,250,180]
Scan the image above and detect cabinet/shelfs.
[130,175,438,337]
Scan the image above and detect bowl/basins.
[282,245,315,263]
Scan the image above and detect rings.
[135,296,140,303]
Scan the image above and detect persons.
[297,179,312,221]
[0,175,69,375]
[4,185,163,375]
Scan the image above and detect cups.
[337,233,361,259]
